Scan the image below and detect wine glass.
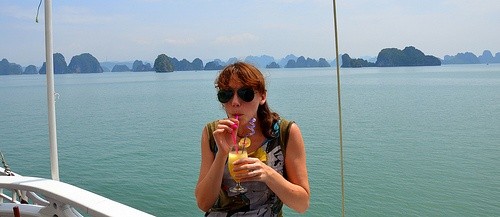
[228,151,249,192]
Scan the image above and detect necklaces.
[237,134,245,138]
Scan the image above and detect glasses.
[217,86,259,103]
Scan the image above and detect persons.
[195,62,310,217]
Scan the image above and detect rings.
[253,171,256,177]
